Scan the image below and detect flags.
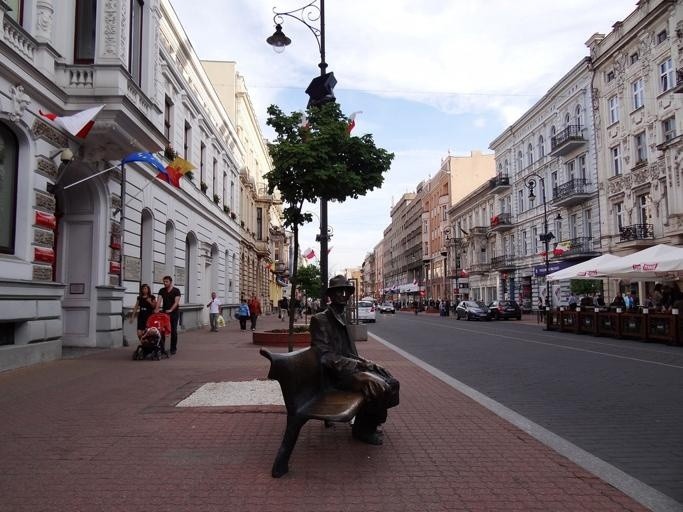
[153,155,198,189]
[37,101,106,140]
[302,248,315,263]
[346,109,364,134]
[120,151,171,184]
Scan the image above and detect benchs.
[258,346,363,478]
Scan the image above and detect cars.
[348,298,395,325]
[485,299,522,321]
[307,295,330,312]
[454,299,492,322]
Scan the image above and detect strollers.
[130,312,173,361]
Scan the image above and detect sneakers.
[170,347,177,355]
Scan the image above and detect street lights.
[409,248,423,303]
[441,222,459,318]
[263,0,336,314]
[522,172,555,312]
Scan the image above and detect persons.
[277,295,332,323]
[391,295,461,320]
[154,275,182,355]
[128,283,156,343]
[206,292,221,332]
[308,274,400,447]
[141,326,160,344]
[249,296,262,331]
[567,283,683,325]
[236,299,250,331]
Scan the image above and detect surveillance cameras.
[61,149,74,166]
